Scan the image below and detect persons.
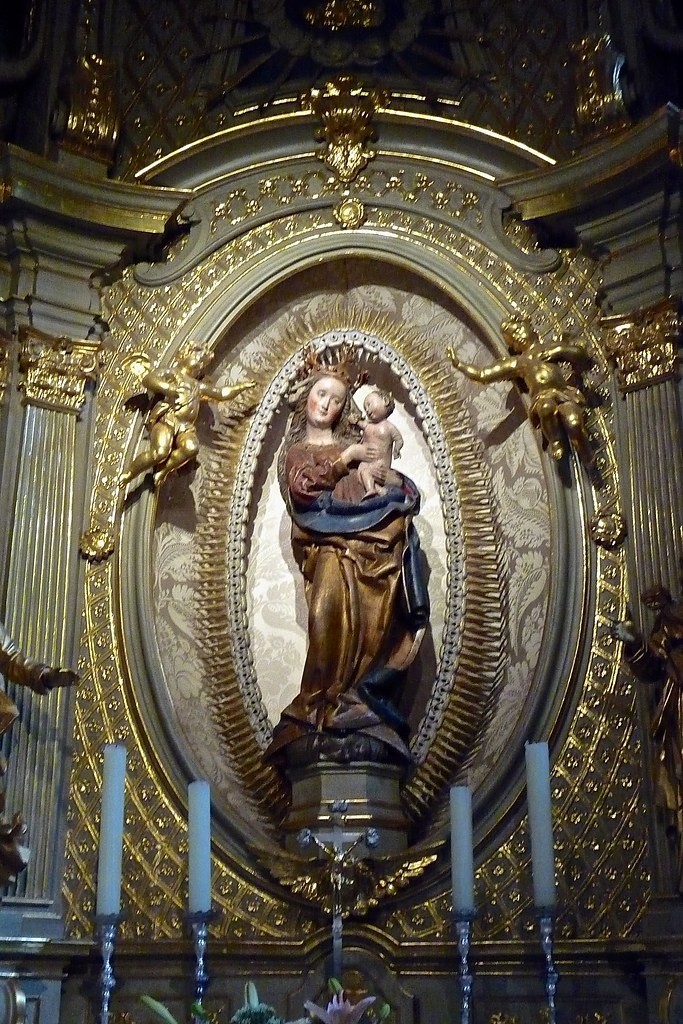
[265,371,430,764]
[444,314,597,470]
[348,391,404,500]
[117,340,257,491]
[0,622,81,777]
[310,835,363,914]
[608,586,683,833]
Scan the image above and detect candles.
[449,784,475,911]
[525,736,557,909]
[187,779,211,913]
[97,743,126,916]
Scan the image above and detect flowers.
[142,978,391,1024]
